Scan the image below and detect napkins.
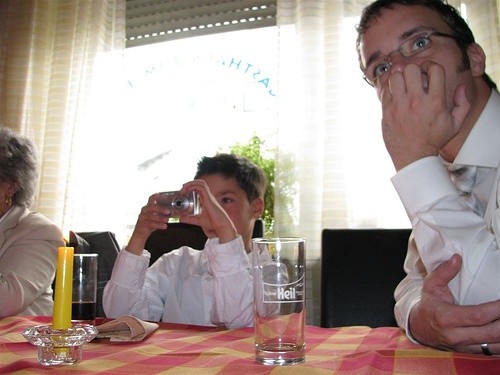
[95,314,160,344]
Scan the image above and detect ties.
[448,166,482,216]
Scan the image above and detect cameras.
[156,190,200,219]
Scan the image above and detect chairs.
[63,231,125,320]
[144,219,263,268]
[319,228,415,328]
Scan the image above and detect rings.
[481,343,493,356]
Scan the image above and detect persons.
[0,128,66,317]
[103,153,289,330]
[357,0,500,354]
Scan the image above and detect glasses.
[363,30,458,88]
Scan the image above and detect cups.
[71,254,99,323]
[251,237,306,366]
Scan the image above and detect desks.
[0,312,500,375]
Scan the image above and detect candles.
[51,238,76,355]
